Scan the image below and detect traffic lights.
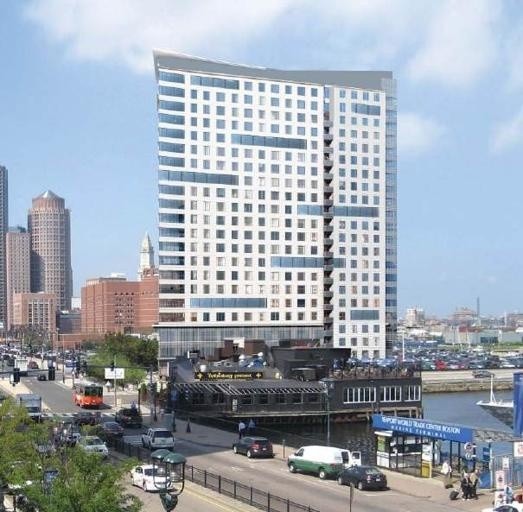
[109,360,114,371]
[150,384,155,394]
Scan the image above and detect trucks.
[13,359,29,376]
[15,393,42,423]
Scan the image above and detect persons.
[441,456,523,506]
[238,420,246,439]
[105,381,111,392]
[131,402,136,409]
[248,419,255,436]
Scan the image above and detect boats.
[474,374,513,430]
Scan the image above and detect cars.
[398,335,521,369]
[127,466,173,494]
[0,343,84,371]
[232,435,275,459]
[334,464,389,492]
[35,373,46,382]
[481,502,522,512]
[25,410,124,497]
[470,370,496,379]
[4,461,42,493]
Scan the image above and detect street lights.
[55,325,60,370]
[150,448,186,511]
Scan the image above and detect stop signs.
[497,476,503,483]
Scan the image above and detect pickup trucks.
[114,407,144,428]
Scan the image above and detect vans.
[286,444,362,482]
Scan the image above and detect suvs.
[139,427,175,451]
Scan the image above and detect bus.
[71,381,105,409]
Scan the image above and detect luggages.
[450,491,459,501]
